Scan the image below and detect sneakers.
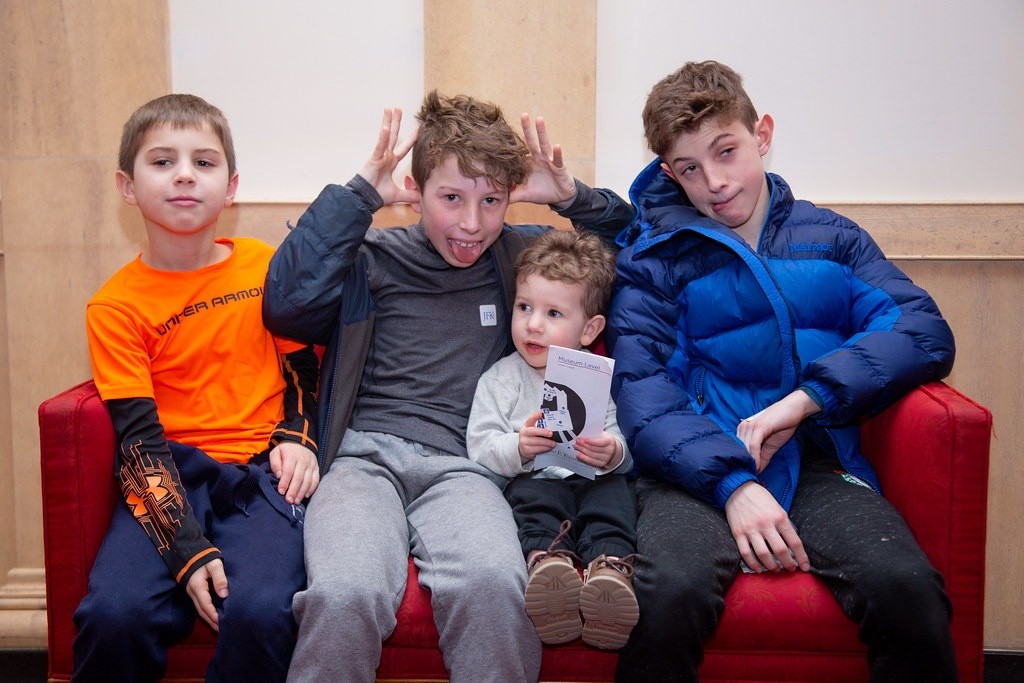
[578,552,640,650]
[523,518,583,644]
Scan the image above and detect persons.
[466,230,641,651]
[261,88,638,682]
[605,60,956,683]
[71,94,320,683]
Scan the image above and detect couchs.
[38,378,993,682]
